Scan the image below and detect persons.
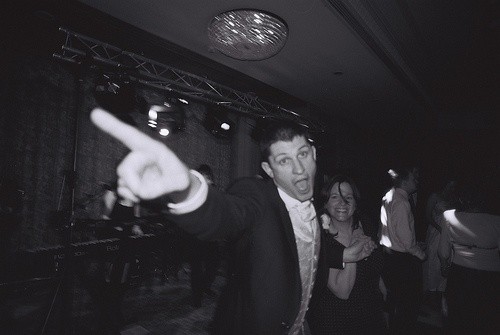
[90,107,377,335]
[426,175,462,308]
[439,175,500,335]
[100,178,142,295]
[378,169,425,335]
[306,174,389,335]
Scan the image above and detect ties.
[408,195,416,238]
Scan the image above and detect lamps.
[207,8,289,61]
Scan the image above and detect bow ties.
[296,200,316,222]
